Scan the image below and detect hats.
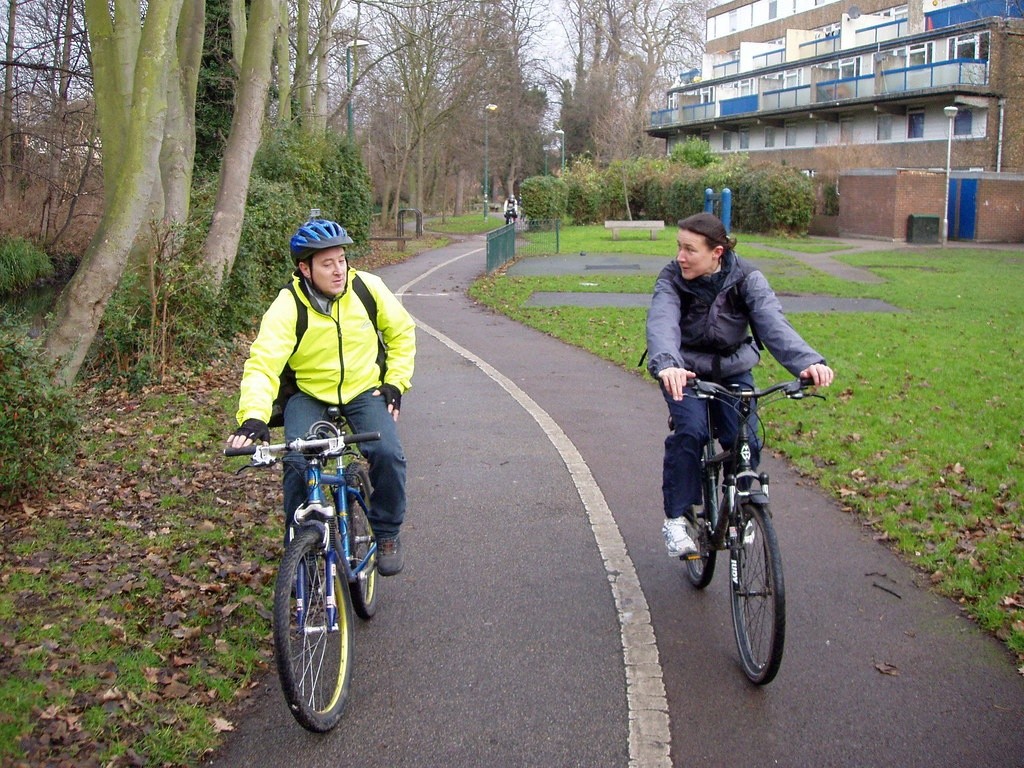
[290,219,354,267]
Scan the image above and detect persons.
[646,213,834,558]
[225,222,420,603]
[503,194,517,226]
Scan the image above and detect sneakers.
[744,520,755,544]
[661,517,697,557]
[376,536,405,576]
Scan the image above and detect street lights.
[938,106,958,245]
[483,103,498,222]
[555,129,567,177]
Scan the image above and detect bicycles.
[667,374,827,684]
[505,213,516,226]
[223,406,383,735]
